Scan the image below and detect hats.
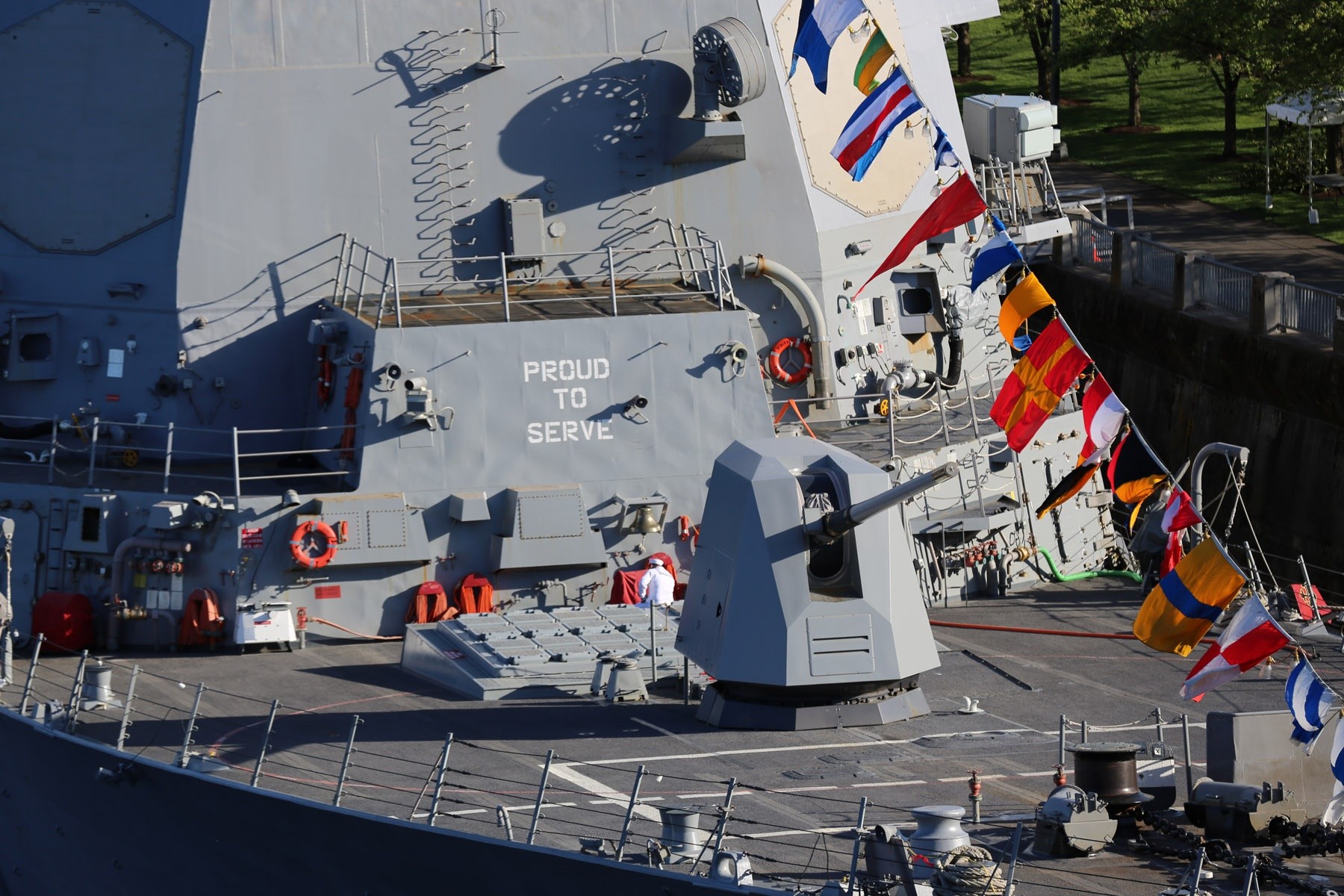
[648,558,664,566]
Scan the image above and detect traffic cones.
[1088,236,1104,263]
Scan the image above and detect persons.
[638,558,675,605]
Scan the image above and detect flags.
[793,0,865,94]
[830,64,925,183]
[1034,372,1128,520]
[1161,485,1205,578]
[1285,653,1338,757]
[1107,428,1169,536]
[854,28,896,97]
[970,215,1023,294]
[990,314,1093,454]
[1179,593,1291,703]
[999,272,1056,352]
[1132,534,1247,658]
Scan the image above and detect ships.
[1,0,1344,896]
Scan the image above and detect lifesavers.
[292,521,337,570]
[318,359,331,406]
[770,335,812,383]
